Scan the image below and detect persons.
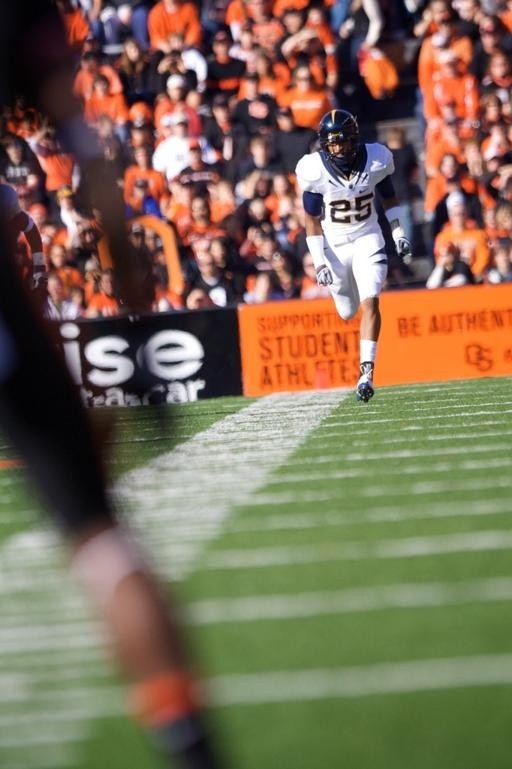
[1,0,231,765]
[1,2,511,318]
[291,108,415,403]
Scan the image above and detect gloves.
[315,264,334,286]
[396,236,411,257]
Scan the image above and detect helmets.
[317,109,360,163]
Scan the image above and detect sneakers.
[356,373,375,403]
